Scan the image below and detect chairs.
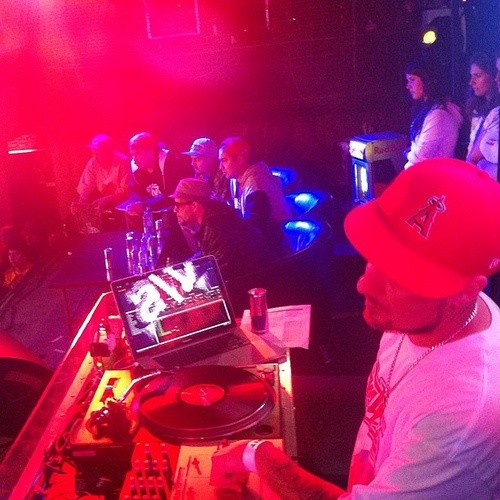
[268,164,333,287]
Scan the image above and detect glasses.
[169,202,193,210]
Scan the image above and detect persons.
[78,132,292,314]
[401,56,500,308]
[208,156,500,500]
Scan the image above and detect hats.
[169,179,210,201]
[181,138,220,158]
[344,158,500,299]
[85,135,112,150]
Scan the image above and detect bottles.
[124,206,165,277]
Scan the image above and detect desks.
[45,227,169,342]
[0,291,297,500]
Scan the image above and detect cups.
[248,286,270,332]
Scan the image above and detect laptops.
[110,255,287,376]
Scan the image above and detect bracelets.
[245,440,273,472]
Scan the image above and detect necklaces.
[383,299,477,410]
